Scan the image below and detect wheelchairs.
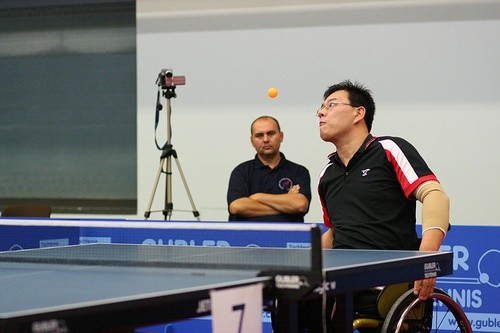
[269,223,473,333]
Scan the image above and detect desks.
[0,218,455,333]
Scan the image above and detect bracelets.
[421,227,447,239]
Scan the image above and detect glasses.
[315,101,359,116]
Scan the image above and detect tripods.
[144,88,201,221]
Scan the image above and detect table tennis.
[267,87,279,98]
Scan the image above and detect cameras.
[158,67,186,88]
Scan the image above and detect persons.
[227,115,312,223]
[270,79,450,333]
